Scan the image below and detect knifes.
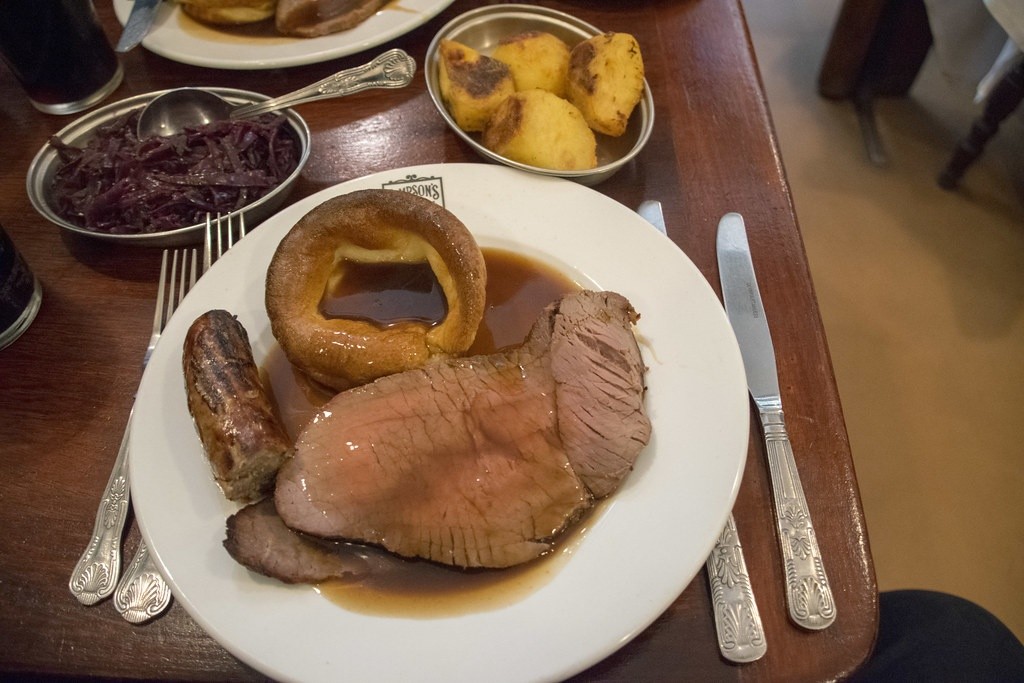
[716,210,836,630]
[112,0,162,53]
[637,199,767,663]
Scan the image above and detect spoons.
[137,48,417,142]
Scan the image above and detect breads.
[438,28,644,171]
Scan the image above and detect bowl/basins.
[26,86,312,250]
[424,3,655,189]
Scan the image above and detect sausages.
[182,307,297,501]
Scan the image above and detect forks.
[67,249,199,607]
[113,211,248,624]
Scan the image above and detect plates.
[112,0,455,70]
[128,162,751,683]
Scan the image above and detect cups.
[0,0,125,115]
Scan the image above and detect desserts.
[265,188,487,389]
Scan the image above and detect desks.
[0,1,880,683]
